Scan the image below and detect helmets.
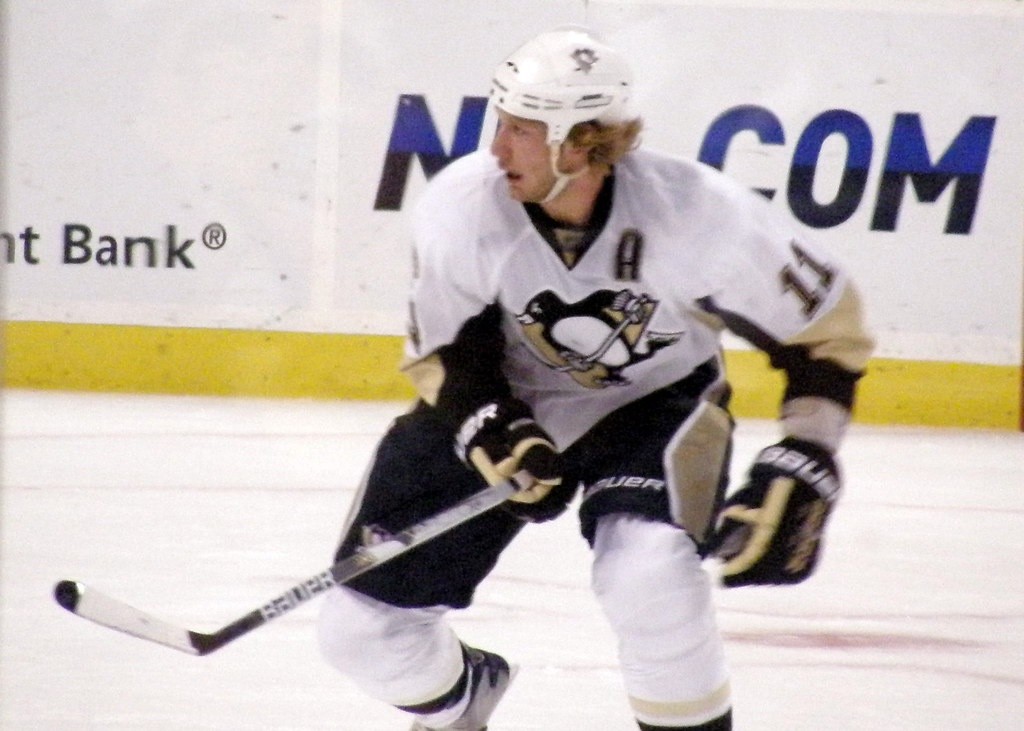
[488,30,641,146]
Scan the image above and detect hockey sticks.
[51,464,536,656]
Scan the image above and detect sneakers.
[395,640,520,731]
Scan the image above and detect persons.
[316,27,879,731]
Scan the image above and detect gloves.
[450,382,584,523]
[701,436,840,587]
